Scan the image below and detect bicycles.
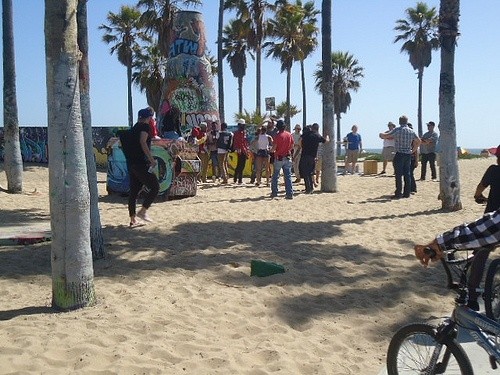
[472,195,500,322]
[386,247,500,375]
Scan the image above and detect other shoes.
[136,212,153,222]
[130,222,146,228]
[466,301,480,311]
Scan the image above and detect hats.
[293,124,300,129]
[238,118,245,124]
[426,121,435,126]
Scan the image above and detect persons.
[379,115,500,269]
[128,107,363,228]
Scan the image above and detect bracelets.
[380,132,382,137]
